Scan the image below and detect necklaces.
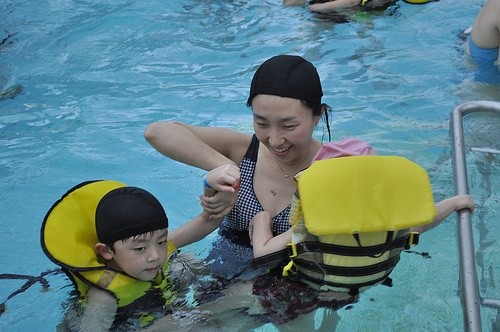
[276,157,308,178]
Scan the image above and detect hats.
[250,55,322,104]
[95,186,168,243]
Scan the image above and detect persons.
[282,0,437,12]
[468,0,500,61]
[145,55,381,237]
[248,156,476,260]
[40,164,241,290]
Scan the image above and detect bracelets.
[202,178,213,188]
